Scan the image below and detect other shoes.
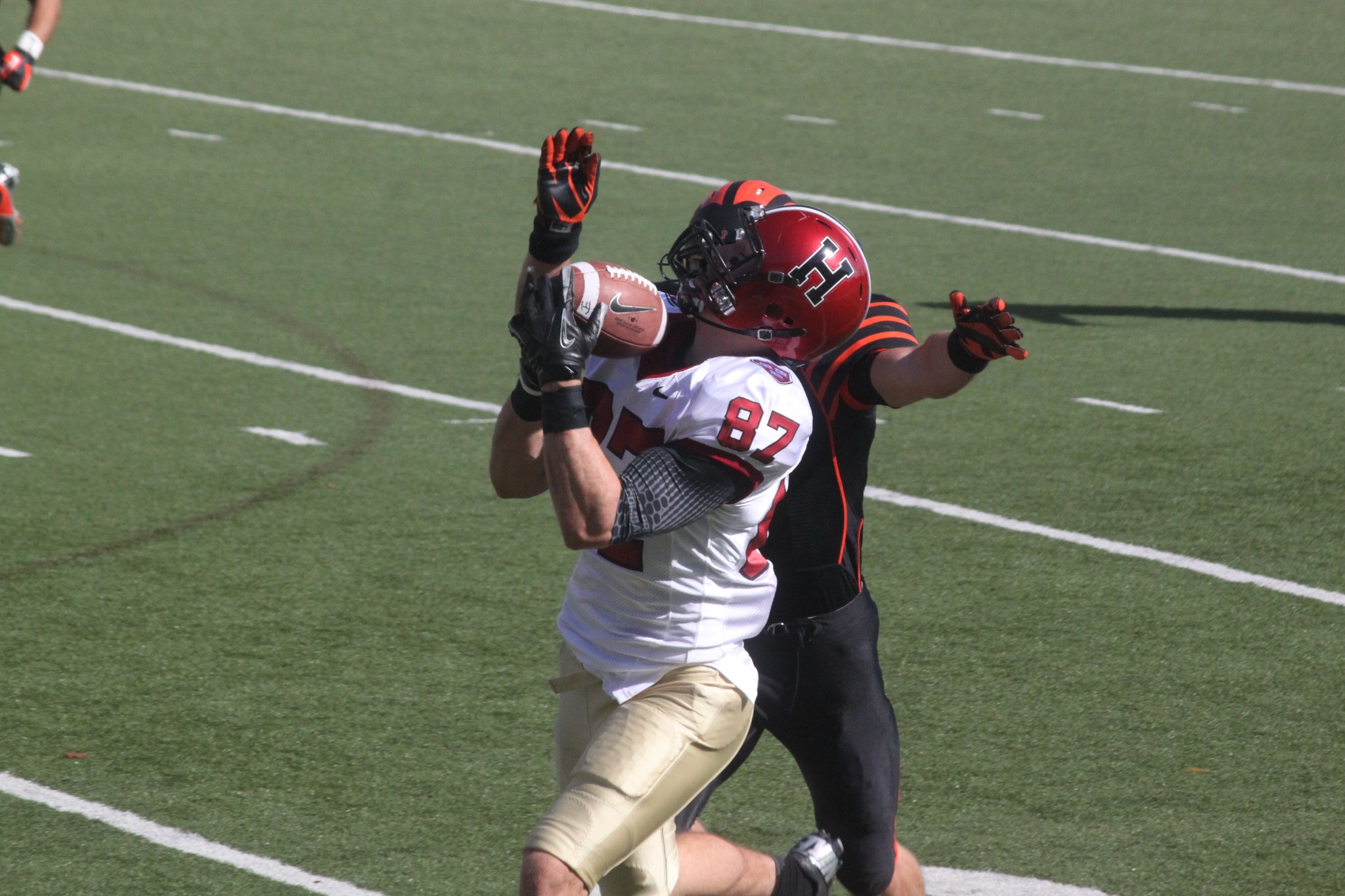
[0,182,21,246]
[786,829,844,896]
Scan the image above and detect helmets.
[688,180,797,226]
[704,207,872,362]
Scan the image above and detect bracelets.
[540,385,589,434]
[511,385,542,421]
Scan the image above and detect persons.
[489,203,869,896]
[515,125,1028,896]
[0,0,61,247]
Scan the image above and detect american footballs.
[549,261,668,355]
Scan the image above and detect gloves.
[506,266,608,422]
[946,290,1029,372]
[529,126,600,263]
[0,53,35,92]
